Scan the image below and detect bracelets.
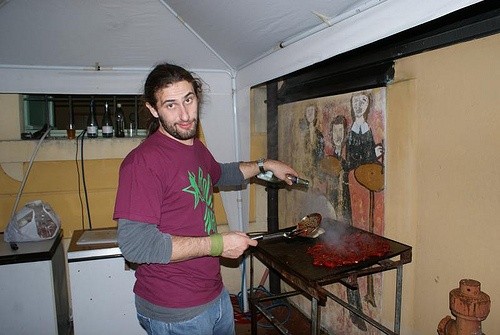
[209,234,225,256]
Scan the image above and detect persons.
[113,63,301,335]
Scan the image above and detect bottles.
[101,101,114,137]
[86,100,99,138]
[114,102,125,138]
[253,170,310,187]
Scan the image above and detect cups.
[127,121,138,138]
[66,129,75,139]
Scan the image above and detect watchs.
[256,159,267,174]
